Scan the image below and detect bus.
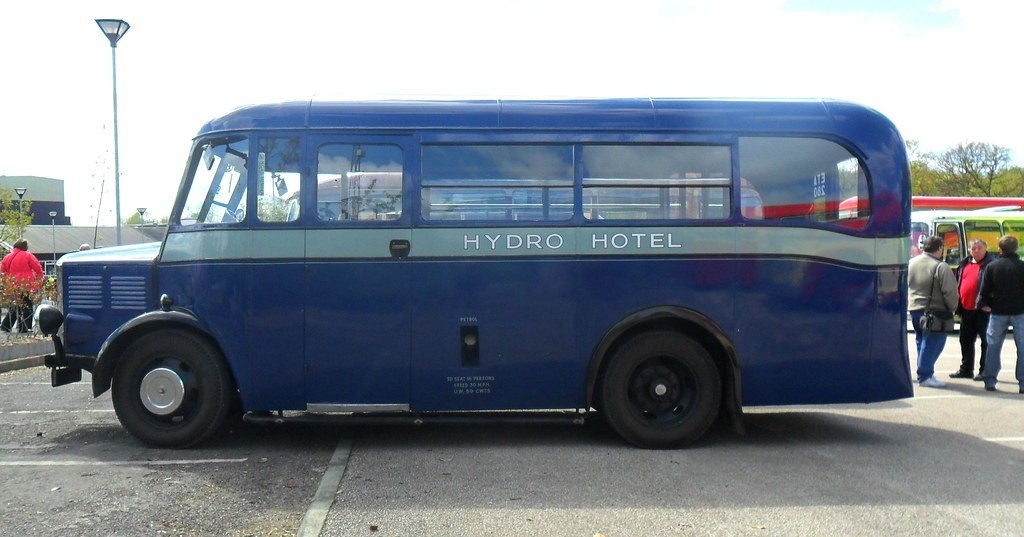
[838,193,1024,277]
[910,211,1024,324]
[34,99,915,452]
[741,197,901,233]
[287,172,704,221]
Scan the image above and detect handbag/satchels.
[919,310,955,333]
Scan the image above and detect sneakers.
[918,376,948,388]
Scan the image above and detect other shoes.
[984,386,996,391]
[1018,384,1024,391]
[18,330,31,334]
[0,325,13,333]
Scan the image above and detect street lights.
[94,16,133,248]
[14,188,26,237]
[48,212,58,276]
[137,208,147,246]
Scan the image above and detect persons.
[908,236,959,387]
[975,236,1024,393]
[0,238,44,332]
[954,238,996,380]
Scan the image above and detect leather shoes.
[974,372,985,381]
[950,370,974,379]
